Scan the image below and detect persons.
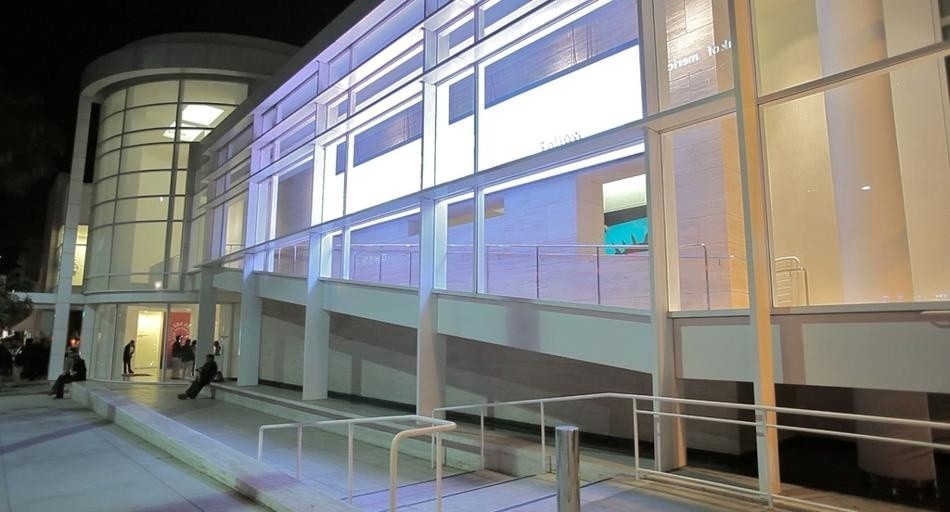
[123,339,136,376]
[171,334,182,380]
[182,338,193,378]
[177,353,218,401]
[47,354,87,401]
[0,342,13,396]
[20,337,38,383]
[190,339,199,376]
[214,341,221,355]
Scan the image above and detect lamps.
[69,330,81,348]
